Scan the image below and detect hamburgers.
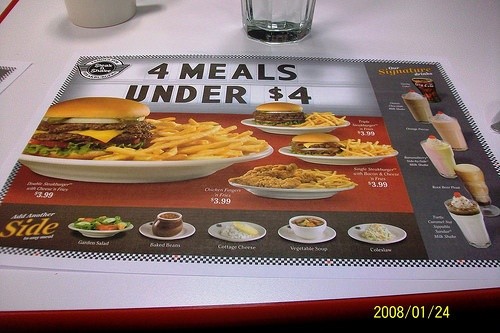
[251,103,307,127]
[23,97,150,160]
[288,133,343,156]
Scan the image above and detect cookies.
[233,223,258,234]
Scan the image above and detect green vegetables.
[74,216,131,230]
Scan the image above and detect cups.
[152,212,183,237]
[239,0,316,45]
[403,97,433,122]
[65,0,136,27]
[453,167,500,217]
[431,116,468,151]
[420,139,458,178]
[445,201,491,250]
[412,78,440,102]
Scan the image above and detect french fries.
[289,112,346,127]
[336,139,396,156]
[93,117,269,161]
[297,169,359,188]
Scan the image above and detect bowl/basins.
[289,214,327,238]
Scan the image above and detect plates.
[228,182,356,199]
[278,225,337,243]
[347,223,407,245]
[69,223,133,237]
[279,146,399,166]
[139,222,196,241]
[17,146,272,182]
[208,221,267,243]
[241,118,350,135]
